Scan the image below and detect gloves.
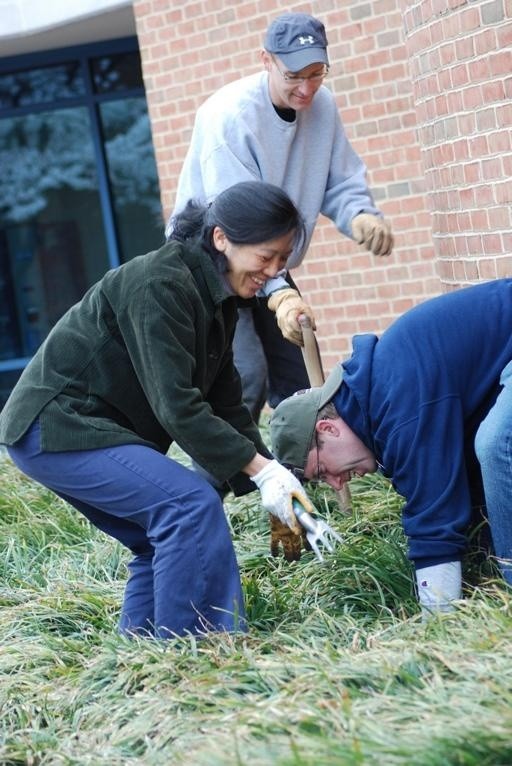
[351,212,394,257]
[249,458,316,537]
[266,509,315,562]
[267,288,316,348]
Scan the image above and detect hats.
[262,13,331,73]
[267,361,346,481]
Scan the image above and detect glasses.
[309,430,324,484]
[270,52,329,85]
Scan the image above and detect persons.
[163,8,397,512]
[267,275,511,625]
[0,179,321,638]
